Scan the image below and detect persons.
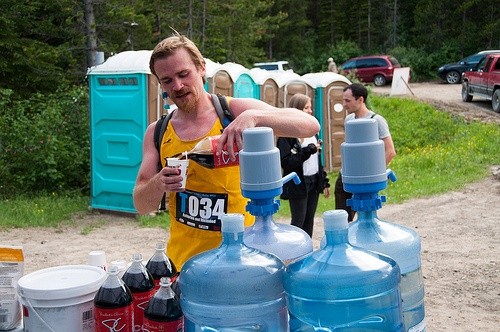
[277,94,331,239]
[334,83,395,223]
[133,33,320,274]
[326,57,336,72]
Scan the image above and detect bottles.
[142,277,184,332]
[146,241,178,293]
[93,266,133,332]
[121,254,154,332]
[87,250,107,272]
[182,135,243,169]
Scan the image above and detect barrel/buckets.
[179,213,289,332]
[17,264,109,332]
[243,215,313,264]
[283,210,406,332]
[320,210,426,332]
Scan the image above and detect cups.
[166,158,189,191]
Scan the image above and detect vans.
[254,61,294,74]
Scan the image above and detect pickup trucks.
[460,54,500,113]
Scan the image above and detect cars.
[334,55,403,88]
[436,49,500,85]
